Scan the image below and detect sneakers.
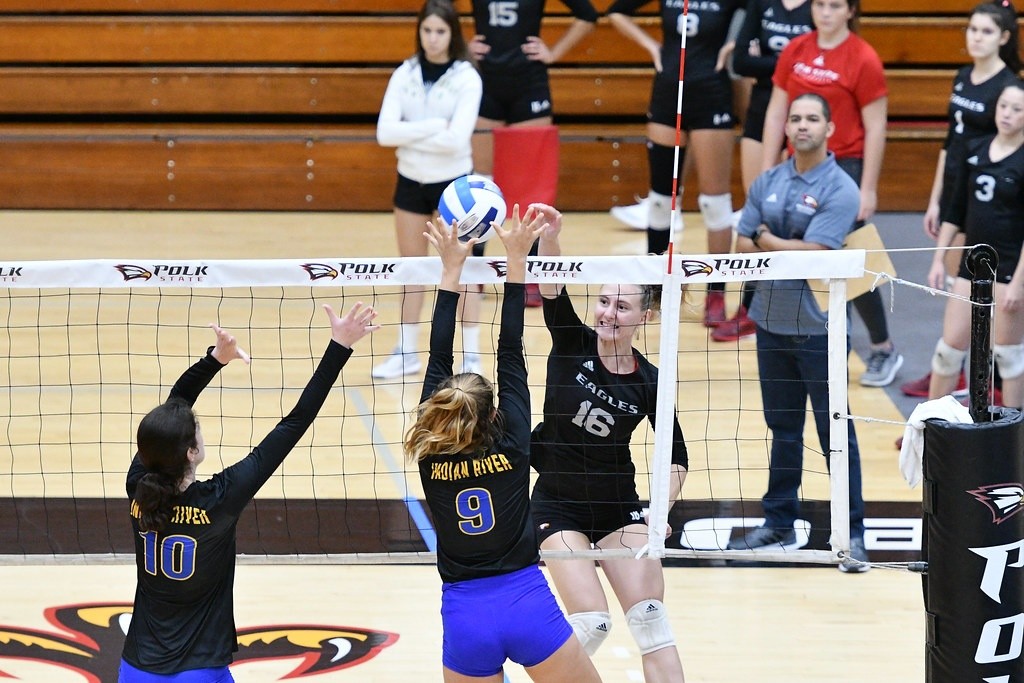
[959,382,1002,407]
[370,347,421,379]
[859,342,904,387]
[459,357,482,376]
[712,306,755,342]
[900,369,969,397]
[524,283,543,307]
[704,289,726,327]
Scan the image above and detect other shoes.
[731,208,745,230]
[728,522,797,552]
[838,537,871,573]
[610,193,649,230]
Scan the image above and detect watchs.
[751,228,769,248]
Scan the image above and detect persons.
[117,301,382,683]
[373,0,1024,575]
[530,203,689,683]
[404,203,606,683]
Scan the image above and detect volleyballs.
[438,175,508,247]
